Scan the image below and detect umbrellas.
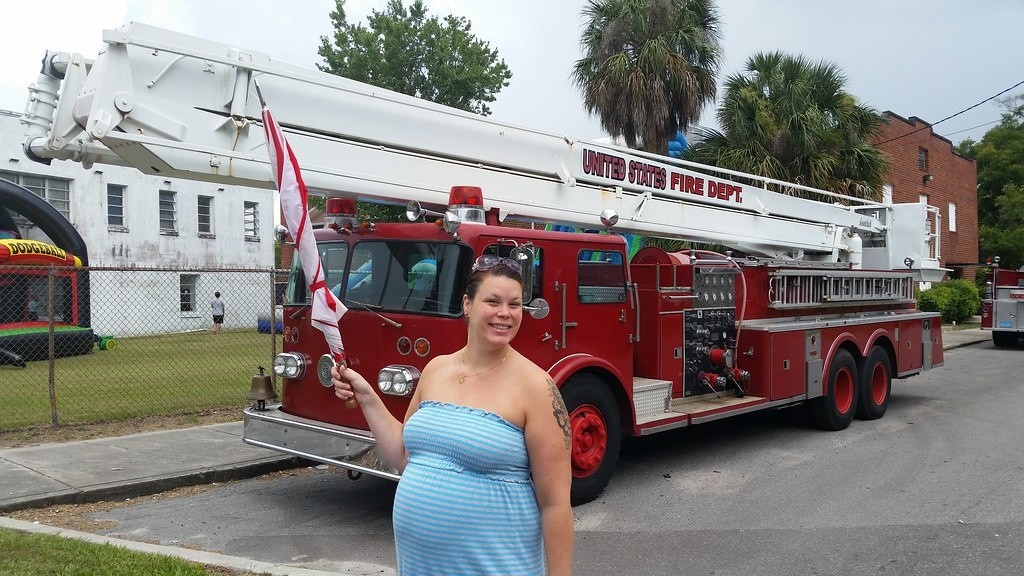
[255,80,360,411]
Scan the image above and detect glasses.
[471,254,522,274]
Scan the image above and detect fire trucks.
[979,255,1024,346]
[20,20,956,513]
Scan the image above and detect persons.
[210,291,225,337]
[330,253,575,576]
[26,294,43,313]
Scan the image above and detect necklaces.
[457,346,512,384]
